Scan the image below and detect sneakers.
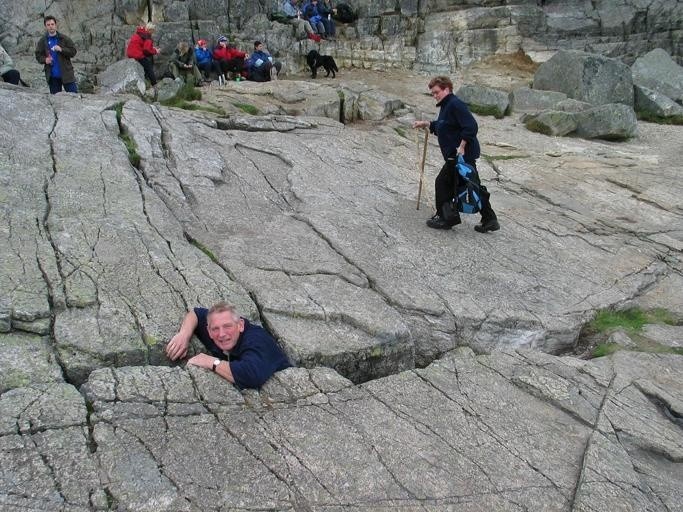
[426,214,451,229]
[197,79,203,86]
[322,32,335,40]
[151,79,157,85]
[474,219,500,233]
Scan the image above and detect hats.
[217,36,227,44]
[197,39,206,46]
[144,22,155,30]
[137,26,147,35]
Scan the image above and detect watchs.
[212,358,222,371]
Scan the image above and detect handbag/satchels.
[160,64,175,80]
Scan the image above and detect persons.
[302,1,328,41]
[282,0,317,40]
[411,76,500,233]
[35,16,77,94]
[167,34,283,83]
[0,45,22,85]
[165,302,291,389]
[318,0,337,42]
[126,20,161,86]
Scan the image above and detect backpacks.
[447,153,490,214]
